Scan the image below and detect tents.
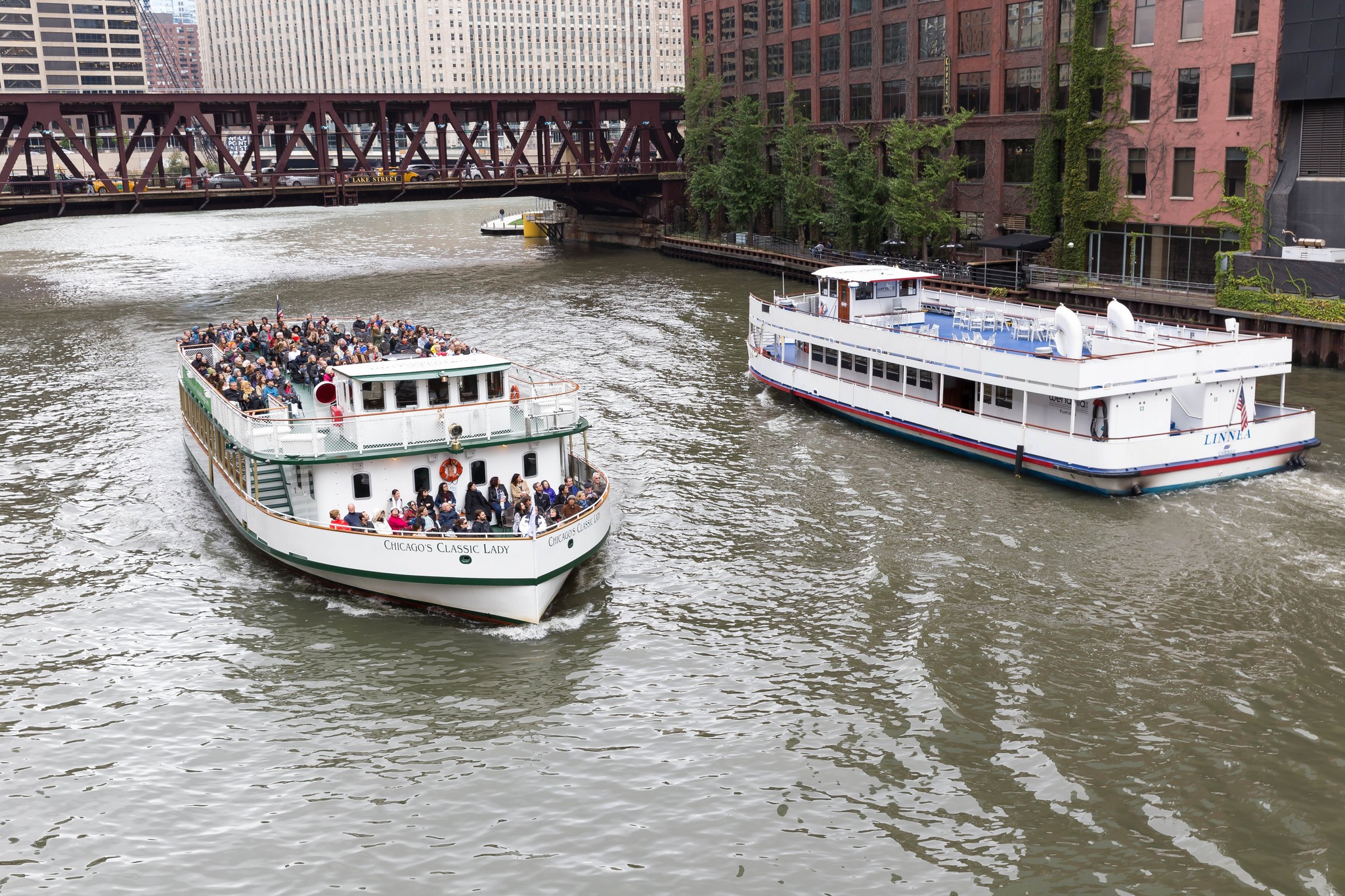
[976,234,1055,291]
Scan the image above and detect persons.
[329,472,606,538]
[463,162,473,181]
[555,162,562,174]
[86,174,99,195]
[814,242,824,257]
[650,156,653,168]
[500,207,504,221]
[827,241,833,250]
[176,314,478,423]
[677,156,682,172]
[573,159,581,177]
[632,156,641,172]
[621,157,632,175]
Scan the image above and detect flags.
[277,294,284,320]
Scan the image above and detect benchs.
[919,301,1094,329]
[436,505,515,529]
[855,310,925,326]
[850,251,870,265]
[822,249,837,262]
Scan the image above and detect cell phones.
[589,493,594,498]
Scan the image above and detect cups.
[502,496,506,500]
[460,508,462,514]
[314,346,316,350]
[522,491,526,494]
[430,355,433,357]
[448,501,452,505]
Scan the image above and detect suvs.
[407,164,439,181]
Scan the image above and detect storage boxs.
[534,397,575,429]
[246,426,327,458]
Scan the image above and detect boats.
[176,295,612,630]
[745,264,1322,498]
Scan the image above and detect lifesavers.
[439,458,463,482]
[819,300,826,314]
[755,347,768,358]
[510,385,520,404]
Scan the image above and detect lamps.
[447,423,464,454]
[435,371,449,383]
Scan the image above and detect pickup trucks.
[9,172,87,196]
[261,165,281,184]
[492,160,528,177]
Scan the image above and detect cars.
[86,177,148,193]
[483,162,501,168]
[30,151,41,155]
[53,149,75,155]
[202,173,258,189]
[280,176,336,187]
[600,161,640,174]
[374,167,421,182]
[498,146,504,150]
[461,166,505,180]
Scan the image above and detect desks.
[961,260,970,265]
[382,354,420,361]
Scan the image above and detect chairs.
[809,248,825,259]
[854,307,1108,353]
[209,324,429,417]
[399,491,582,538]
[867,250,972,279]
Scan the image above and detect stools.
[891,299,908,328]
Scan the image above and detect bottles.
[510,497,512,505]
[457,505,460,513]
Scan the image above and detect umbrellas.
[880,236,907,257]
[940,241,963,248]
